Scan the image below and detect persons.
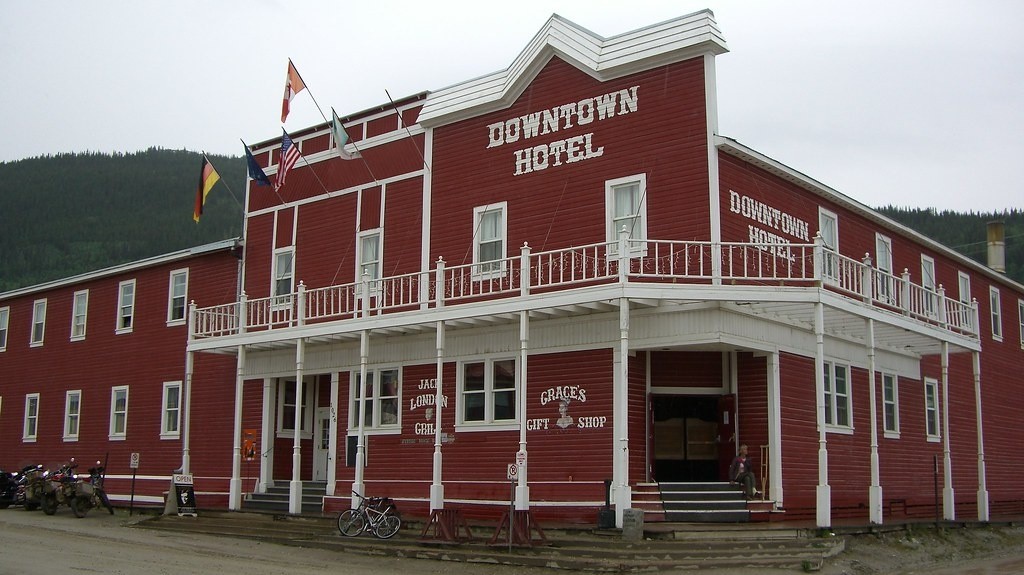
[728,444,763,500]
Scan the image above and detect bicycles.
[335,490,400,538]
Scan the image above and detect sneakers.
[744,495,752,501]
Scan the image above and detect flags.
[273,130,302,193]
[241,140,271,186]
[192,153,221,224]
[281,61,306,123]
[332,110,364,160]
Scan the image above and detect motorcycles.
[0,470,29,509]
[34,457,81,515]
[11,463,49,512]
[70,458,114,519]
